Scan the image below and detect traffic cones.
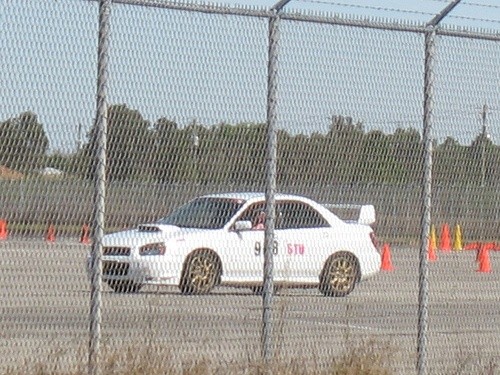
[426,235,438,261]
[465,238,500,272]
[439,224,452,253]
[44,224,57,241]
[0,216,8,239]
[378,243,395,272]
[428,223,439,251]
[79,222,92,243]
[452,225,463,251]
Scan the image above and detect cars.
[88,192,384,299]
